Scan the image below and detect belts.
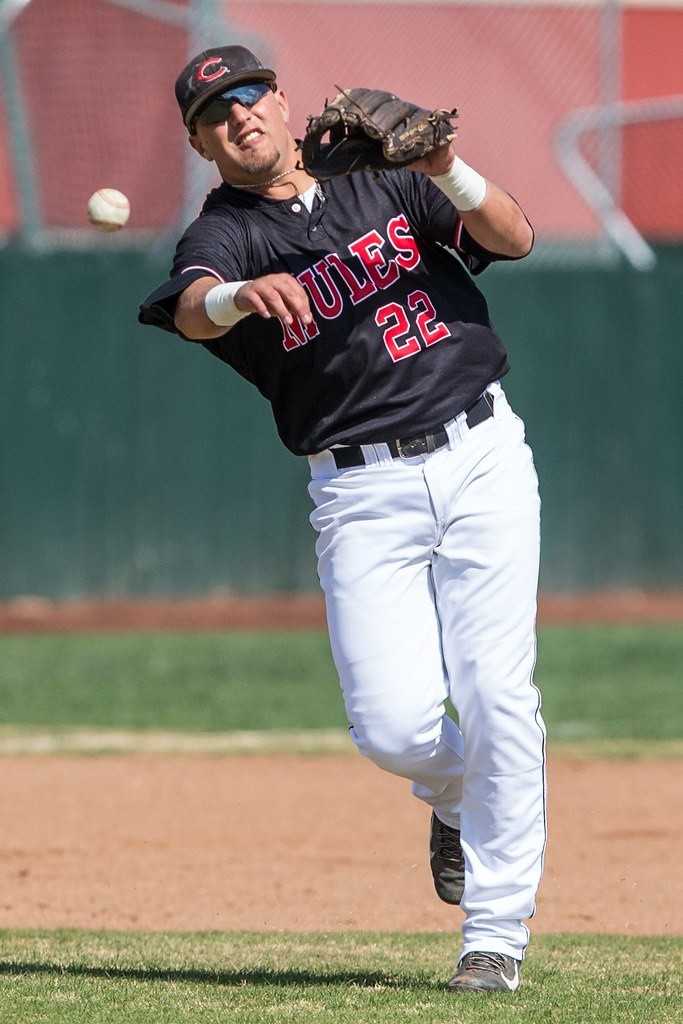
[329,392,493,470]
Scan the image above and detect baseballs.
[86,187,129,233]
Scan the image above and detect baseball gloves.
[300,87,458,180]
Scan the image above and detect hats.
[174,45,276,126]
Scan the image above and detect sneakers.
[445,951,521,992]
[430,809,464,906]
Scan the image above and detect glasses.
[188,81,277,135]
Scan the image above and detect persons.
[138,45,547,994]
